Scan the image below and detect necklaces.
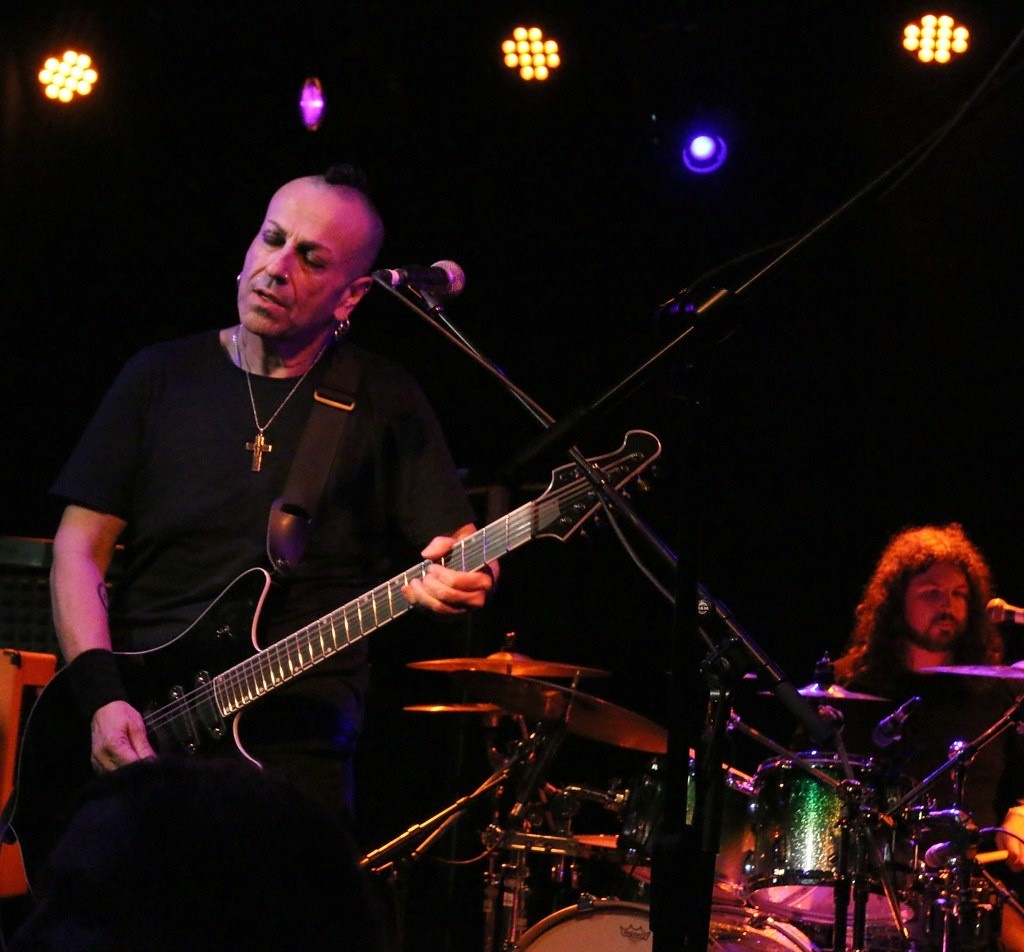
[236,323,332,474]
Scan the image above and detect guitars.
[16,430,663,929]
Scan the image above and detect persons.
[799,522,1024,952]
[46,165,502,952]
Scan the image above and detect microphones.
[985,598,1024,625]
[924,827,996,868]
[373,260,465,302]
[872,696,922,747]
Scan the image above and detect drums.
[493,749,1024,952]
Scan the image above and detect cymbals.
[746,681,890,711]
[920,658,1024,683]
[398,650,674,757]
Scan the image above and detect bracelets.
[481,564,496,593]
